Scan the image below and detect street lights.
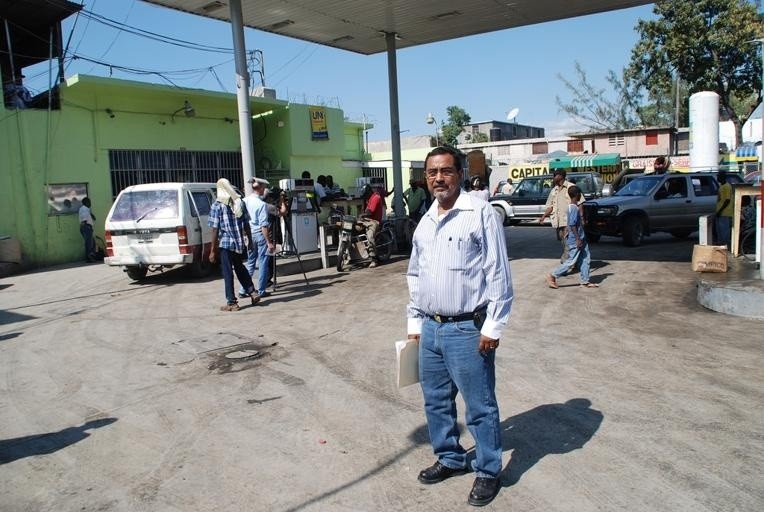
[425,111,442,149]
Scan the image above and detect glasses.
[424,168,464,177]
[553,172,564,178]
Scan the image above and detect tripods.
[272,206,310,292]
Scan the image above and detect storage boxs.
[688,243,731,275]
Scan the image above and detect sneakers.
[579,283,599,289]
[238,281,273,297]
[368,261,378,268]
[220,302,241,311]
[250,291,261,305]
[546,273,560,290]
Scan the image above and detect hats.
[409,178,417,183]
[716,170,729,178]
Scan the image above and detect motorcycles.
[327,202,397,271]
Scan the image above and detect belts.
[424,311,476,324]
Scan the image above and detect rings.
[489,346,495,349]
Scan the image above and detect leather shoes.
[468,474,504,507]
[418,460,469,485]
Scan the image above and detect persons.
[667,182,682,198]
[738,196,757,254]
[405,147,514,507]
[654,156,671,176]
[715,169,734,251]
[208,168,436,312]
[500,167,600,287]
[79,197,97,262]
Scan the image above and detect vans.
[101,182,249,280]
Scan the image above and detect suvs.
[482,168,764,249]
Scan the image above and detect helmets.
[360,183,372,197]
